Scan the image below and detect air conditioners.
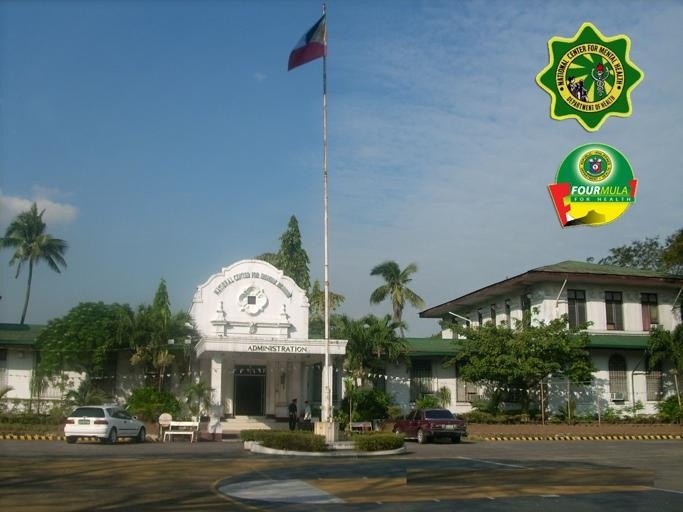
[611,392,625,401]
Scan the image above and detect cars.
[64,405,146,443]
[395,407,467,442]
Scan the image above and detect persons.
[304,401,312,424]
[288,398,297,430]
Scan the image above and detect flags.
[288,14,327,71]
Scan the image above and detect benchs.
[162,421,199,443]
